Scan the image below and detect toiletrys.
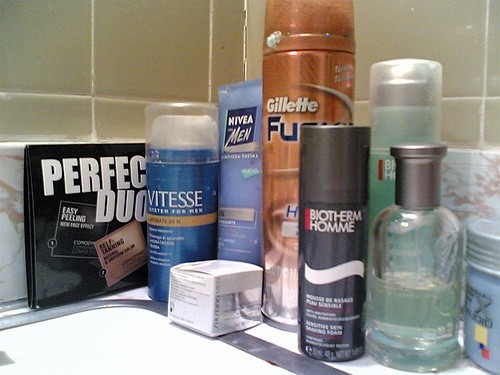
[138,101,224,300]
[460,212,500,375]
[261,0,359,328]
[294,123,371,366]
[165,255,264,341]
[215,74,266,273]
[364,55,446,285]
[365,137,464,375]
